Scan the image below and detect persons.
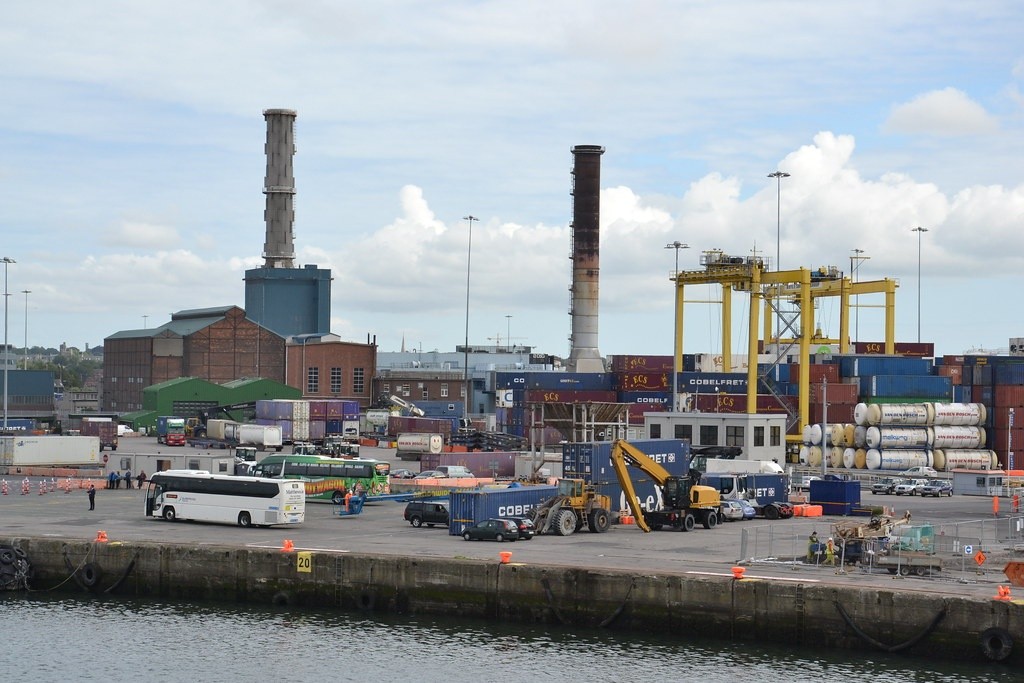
[108,469,146,489]
[87,484,95,510]
[807,531,819,564]
[344,490,352,512]
[823,537,834,566]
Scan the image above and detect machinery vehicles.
[526,477,613,537]
[609,437,725,533]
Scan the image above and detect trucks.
[158,415,207,447]
[79,416,118,452]
[562,438,743,528]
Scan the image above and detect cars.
[720,500,757,522]
[460,515,537,543]
[391,465,476,479]
[898,466,938,480]
[791,475,821,492]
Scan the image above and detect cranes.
[489,333,528,353]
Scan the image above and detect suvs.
[403,500,449,528]
[894,478,929,496]
[921,479,954,498]
[871,478,901,495]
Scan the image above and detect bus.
[139,446,390,529]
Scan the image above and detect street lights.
[0,256,18,430]
[463,214,481,430]
[768,172,790,363]
[142,315,148,329]
[664,240,691,413]
[506,315,512,352]
[21,289,32,370]
[911,226,930,343]
[851,249,864,342]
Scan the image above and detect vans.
[118,424,133,437]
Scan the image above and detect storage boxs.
[0,369,101,466]
[389,343,1024,534]
[256,399,360,442]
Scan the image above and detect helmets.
[829,537,832,540]
